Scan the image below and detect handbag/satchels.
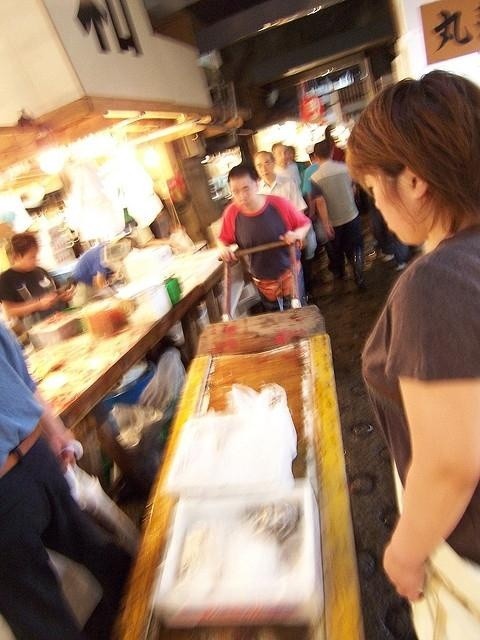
[391,460,480,640]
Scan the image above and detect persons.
[0,233,74,331]
[213,137,410,312]
[70,237,131,299]
[347,69,479,640]
[0,321,132,639]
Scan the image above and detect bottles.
[121,206,139,229]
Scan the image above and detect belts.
[0,421,44,479]
